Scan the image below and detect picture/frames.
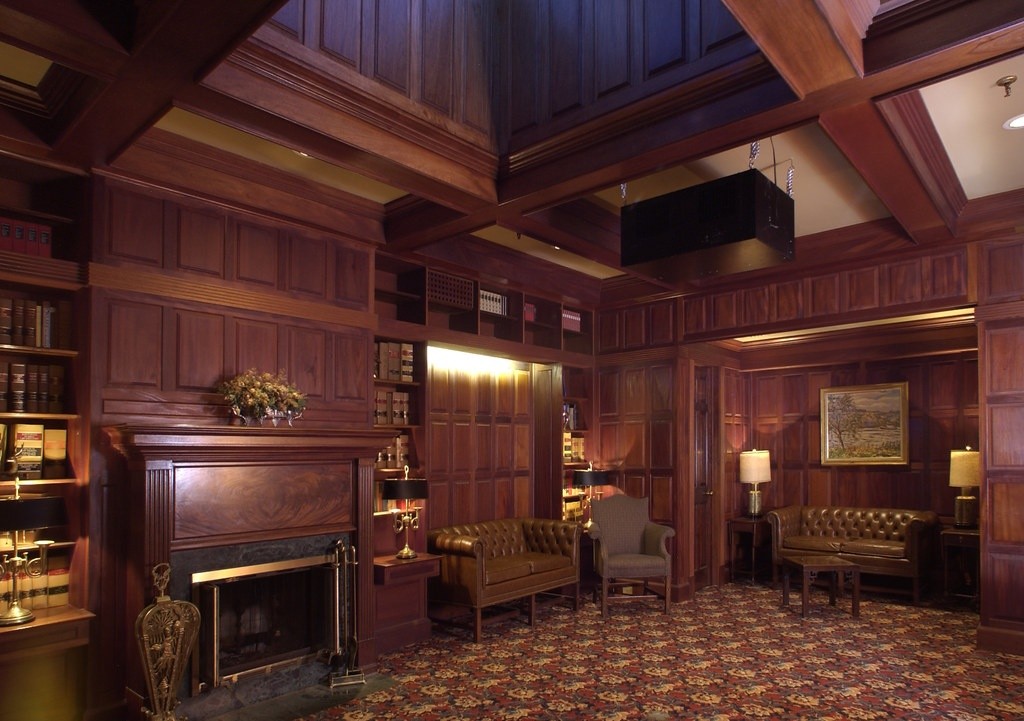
[819,382,909,467]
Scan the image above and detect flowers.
[220,368,308,414]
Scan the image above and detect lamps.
[0,476,69,627]
[948,445,981,529]
[740,447,772,515]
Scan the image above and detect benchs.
[428,518,584,642]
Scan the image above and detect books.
[0,293,68,478]
[479,288,580,332]
[0,216,52,258]
[0,555,70,610]
[562,402,586,522]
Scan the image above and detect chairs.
[584,494,676,616]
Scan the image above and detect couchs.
[767,505,938,606]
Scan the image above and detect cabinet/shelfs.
[0,147,96,721]
[374,250,594,368]
[558,362,597,536]
[372,335,444,586]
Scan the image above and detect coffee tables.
[782,556,860,618]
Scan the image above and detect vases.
[232,405,305,427]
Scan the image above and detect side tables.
[939,525,979,612]
[729,513,769,586]
